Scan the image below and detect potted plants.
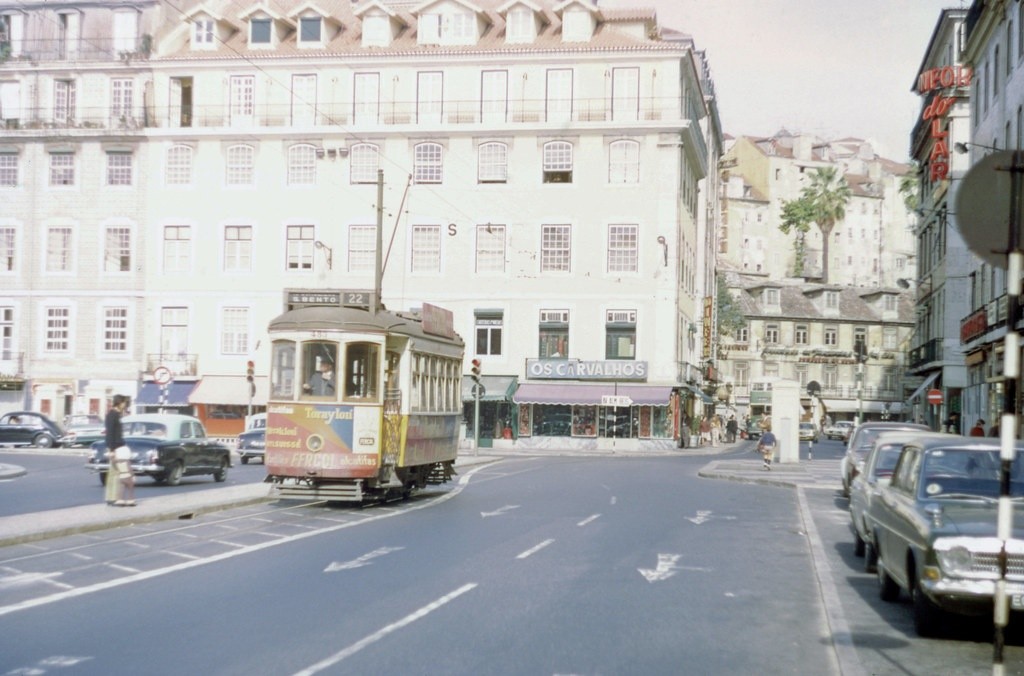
[686,415,702,447]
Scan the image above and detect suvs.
[236,412,267,465]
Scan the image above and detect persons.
[943,412,957,432]
[820,414,831,430]
[757,412,772,434]
[758,427,777,469]
[106,396,135,506]
[700,413,750,444]
[302,362,335,395]
[970,419,986,436]
[987,418,999,437]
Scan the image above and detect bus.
[260,173,465,511]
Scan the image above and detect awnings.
[513,384,672,407]
[687,387,713,404]
[908,370,941,401]
[461,375,516,401]
[135,381,196,406]
[188,376,268,407]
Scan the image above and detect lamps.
[953,142,1003,155]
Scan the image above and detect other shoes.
[108,499,136,506]
[764,463,770,470]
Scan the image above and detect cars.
[537,412,572,436]
[0,412,66,449]
[846,434,925,571]
[824,419,854,441]
[799,422,821,442]
[608,414,634,437]
[63,413,109,447]
[867,435,1024,637]
[82,413,235,488]
[839,421,932,500]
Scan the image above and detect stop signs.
[928,390,943,405]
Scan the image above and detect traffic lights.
[471,358,482,382]
[247,361,256,384]
